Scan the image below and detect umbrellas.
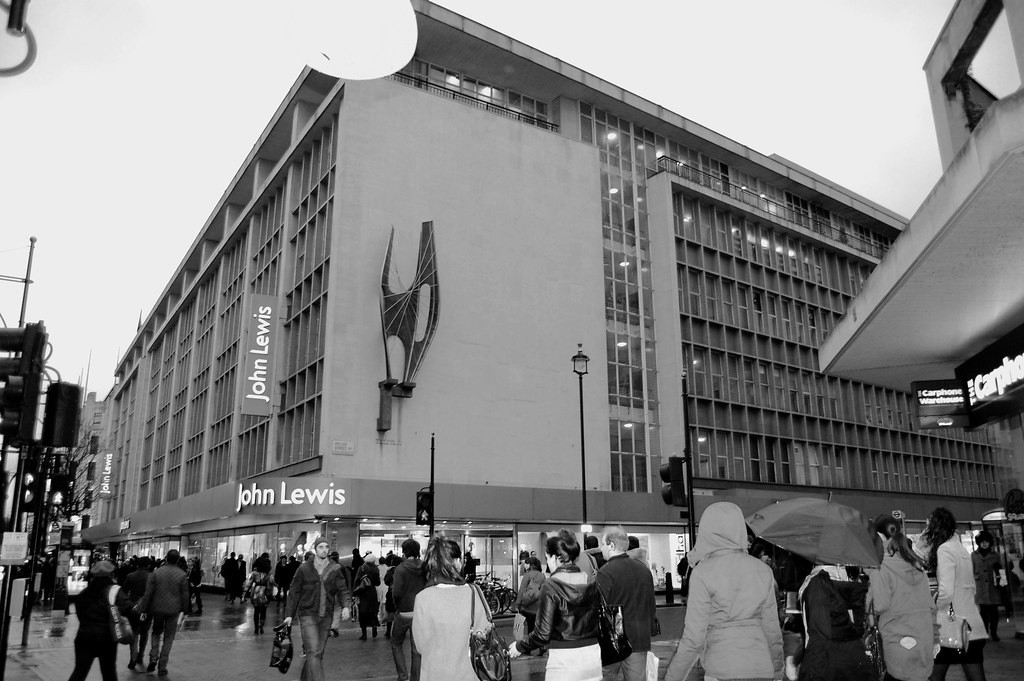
[745,492,884,569]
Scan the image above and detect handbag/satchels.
[353,563,371,595]
[514,606,526,642]
[269,623,293,675]
[265,573,273,602]
[595,578,632,667]
[468,583,514,681]
[109,584,135,645]
[932,592,969,651]
[645,650,660,681]
[860,602,886,678]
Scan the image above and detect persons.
[786,559,878,681]
[283,537,352,681]
[596,527,656,681]
[520,545,529,562]
[329,548,406,640]
[221,553,247,604]
[467,542,476,575]
[925,508,990,681]
[516,536,649,658]
[665,501,786,681]
[413,536,492,681]
[242,553,313,634]
[746,536,811,608]
[391,540,429,681]
[68,549,206,681]
[509,538,604,681]
[866,516,937,681]
[678,553,691,597]
[970,531,1002,641]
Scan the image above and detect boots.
[385,621,393,638]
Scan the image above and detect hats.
[315,537,328,549]
[975,531,996,549]
[365,555,376,565]
[89,560,114,578]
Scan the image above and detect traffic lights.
[0,321,49,448]
[47,472,71,507]
[21,470,44,515]
[659,457,688,507]
[415,491,433,526]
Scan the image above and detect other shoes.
[158,669,168,675]
[128,659,136,670]
[259,625,264,634]
[136,654,144,664]
[147,660,158,673]
[359,634,366,640]
[991,631,1000,642]
[372,626,377,639]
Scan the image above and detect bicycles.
[475,572,519,617]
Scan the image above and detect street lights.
[569,342,590,549]
